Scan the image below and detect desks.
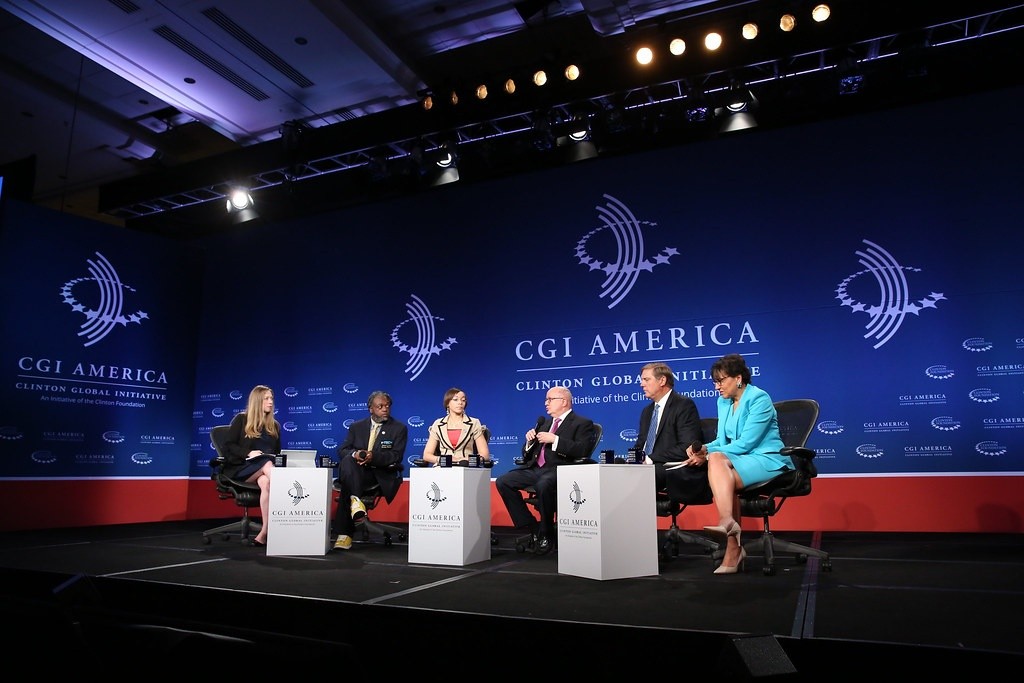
[557,464,659,580]
[266,466,333,557]
[408,466,491,566]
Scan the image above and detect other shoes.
[535,536,554,555]
[249,537,266,547]
[528,527,540,549]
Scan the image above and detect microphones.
[614,457,626,464]
[529,416,545,445]
[692,441,702,453]
[452,460,469,467]
[358,451,367,459]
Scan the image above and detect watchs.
[705,451,710,461]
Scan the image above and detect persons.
[689,356,797,574]
[421,387,490,467]
[333,391,409,549]
[224,384,282,550]
[631,358,707,491]
[494,385,594,547]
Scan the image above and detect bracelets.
[479,455,485,460]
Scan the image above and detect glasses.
[712,376,730,385]
[545,397,563,402]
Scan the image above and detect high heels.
[713,546,746,574]
[702,518,741,547]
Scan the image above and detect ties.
[536,418,561,467]
[367,424,380,455]
[645,404,660,455]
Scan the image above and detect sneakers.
[334,535,353,549]
[350,495,367,520]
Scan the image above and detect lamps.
[422,148,461,190]
[223,189,262,226]
[711,85,760,135]
[553,118,600,163]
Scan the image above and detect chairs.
[202,425,263,546]
[711,398,831,578]
[655,418,719,568]
[331,462,407,547]
[514,422,603,556]
